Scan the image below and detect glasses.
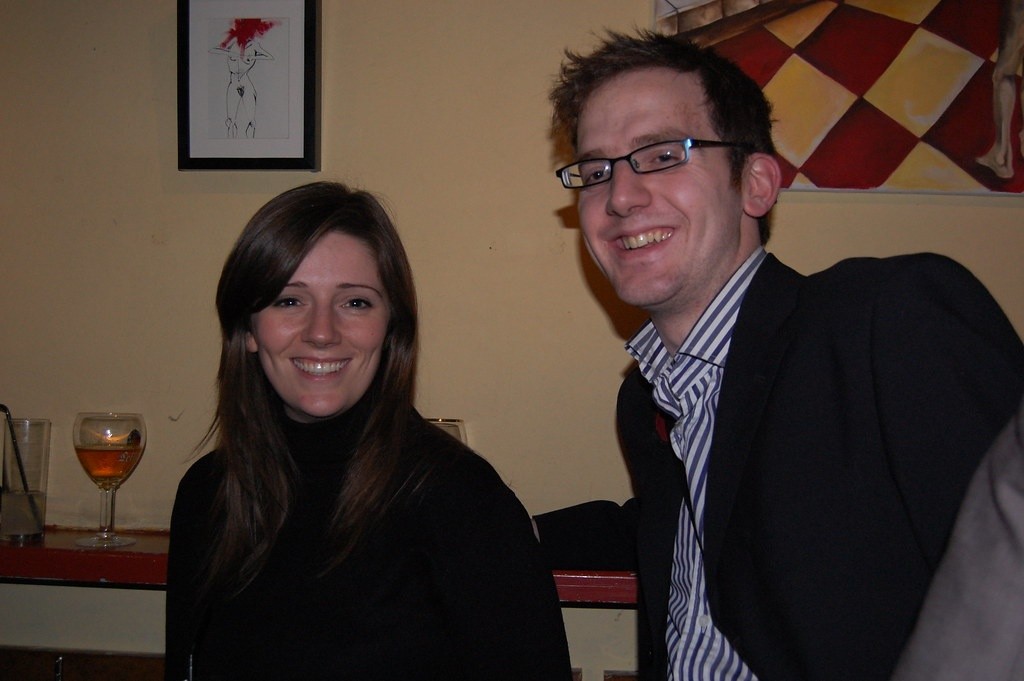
[554,137,754,189]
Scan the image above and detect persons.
[163,180,572,681]
[544,19,1024,681]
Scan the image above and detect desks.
[0,523,639,609]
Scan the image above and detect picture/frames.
[648,0,1023,209]
[177,0,317,171]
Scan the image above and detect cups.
[423,419,467,447]
[2,420,50,542]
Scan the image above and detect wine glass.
[72,412,146,548]
